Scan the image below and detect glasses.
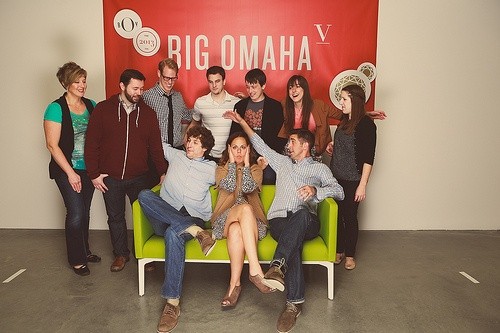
[161,73,178,81]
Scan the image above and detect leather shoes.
[111,255,130,272]
[146,264,155,272]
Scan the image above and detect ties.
[163,94,173,147]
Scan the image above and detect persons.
[139,58,193,148]
[138,125,218,333]
[222,108,345,332]
[226,68,284,185]
[43,61,101,276]
[324,84,377,270]
[277,74,387,165]
[180,66,243,165]
[84,69,168,273]
[210,131,277,309]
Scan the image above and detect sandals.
[248,273,276,293]
[221,286,241,307]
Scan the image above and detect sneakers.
[261,258,285,292]
[277,303,302,333]
[194,231,216,257]
[156,303,180,333]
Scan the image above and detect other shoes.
[345,257,356,269]
[87,255,101,262]
[335,253,343,264]
[72,265,90,276]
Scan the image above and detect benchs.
[133,185,339,301]
